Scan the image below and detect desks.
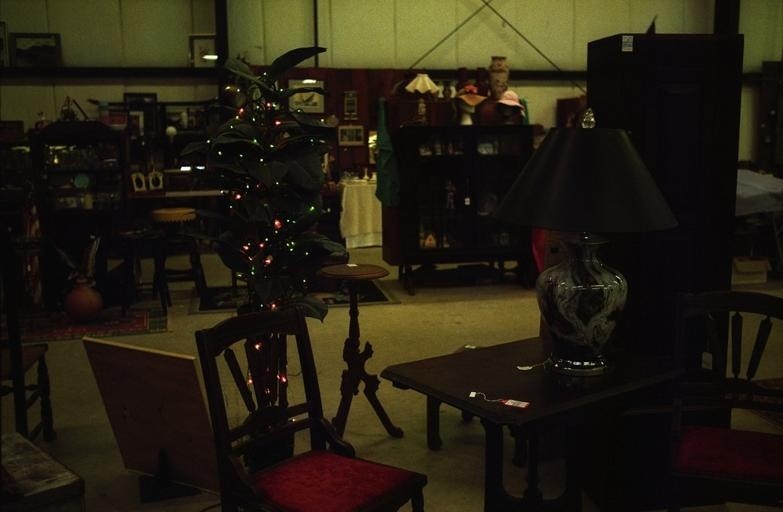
[319,260,406,444]
[1,419,84,511]
[734,168,783,278]
[379,333,682,512]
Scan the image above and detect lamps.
[406,72,444,124]
[497,105,678,379]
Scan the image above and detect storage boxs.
[731,253,771,286]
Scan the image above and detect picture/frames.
[8,32,63,71]
[123,92,157,114]
[188,34,218,66]
[286,76,326,116]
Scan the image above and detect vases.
[489,56,509,100]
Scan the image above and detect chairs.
[1,273,57,442]
[650,290,782,511]
[195,303,427,512]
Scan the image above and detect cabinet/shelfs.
[1,120,546,315]
